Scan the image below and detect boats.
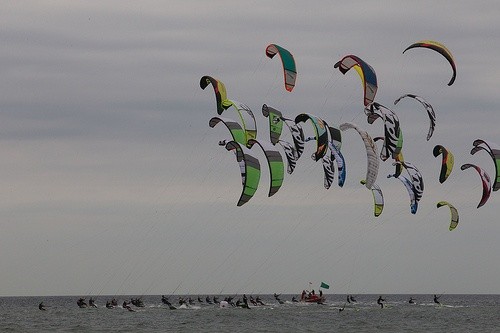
[305,295,324,303]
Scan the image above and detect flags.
[321,282,329,289]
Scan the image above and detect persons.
[224,294,266,309]
[433,294,441,306]
[197,296,204,305]
[346,294,357,304]
[76,297,87,309]
[160,295,174,309]
[88,297,98,308]
[205,297,213,306]
[106,297,145,311]
[213,295,220,306]
[218,140,226,146]
[291,289,323,303]
[409,297,417,305]
[377,295,386,309]
[39,302,47,311]
[273,292,282,303]
[179,297,194,307]
